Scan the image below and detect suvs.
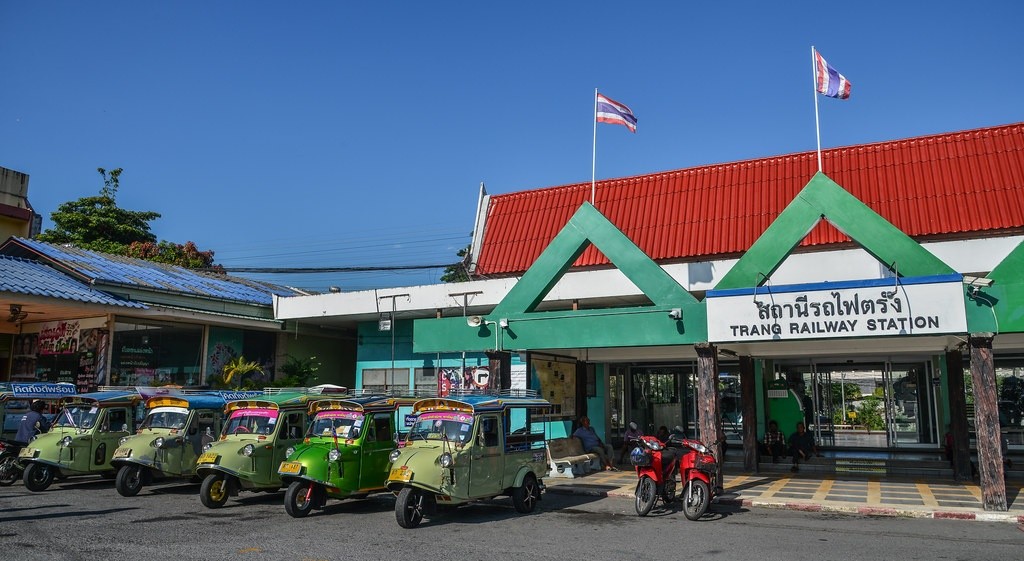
[809,415,835,436]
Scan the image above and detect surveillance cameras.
[669,310,679,319]
[499,320,509,330]
[962,276,996,293]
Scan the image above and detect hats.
[675,426,684,433]
[629,422,637,430]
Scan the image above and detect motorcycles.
[384,386,553,531]
[0,419,58,485]
[109,387,245,496]
[625,419,684,516]
[19,390,165,492]
[276,387,439,517]
[0,379,81,447]
[194,388,375,508]
[669,425,728,519]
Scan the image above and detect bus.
[618,364,810,450]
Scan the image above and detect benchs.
[545,437,599,478]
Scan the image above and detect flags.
[595,93,637,135]
[814,51,852,100]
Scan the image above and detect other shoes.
[605,465,622,472]
[804,454,812,461]
[791,467,799,473]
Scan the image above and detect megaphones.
[467,316,486,327]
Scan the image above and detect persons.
[786,422,816,464]
[720,426,728,463]
[761,419,785,464]
[672,425,687,441]
[619,421,645,469]
[651,425,672,442]
[14,399,55,447]
[943,423,955,465]
[6,319,103,389]
[1000,427,1012,481]
[567,415,622,472]
[510,426,526,434]
